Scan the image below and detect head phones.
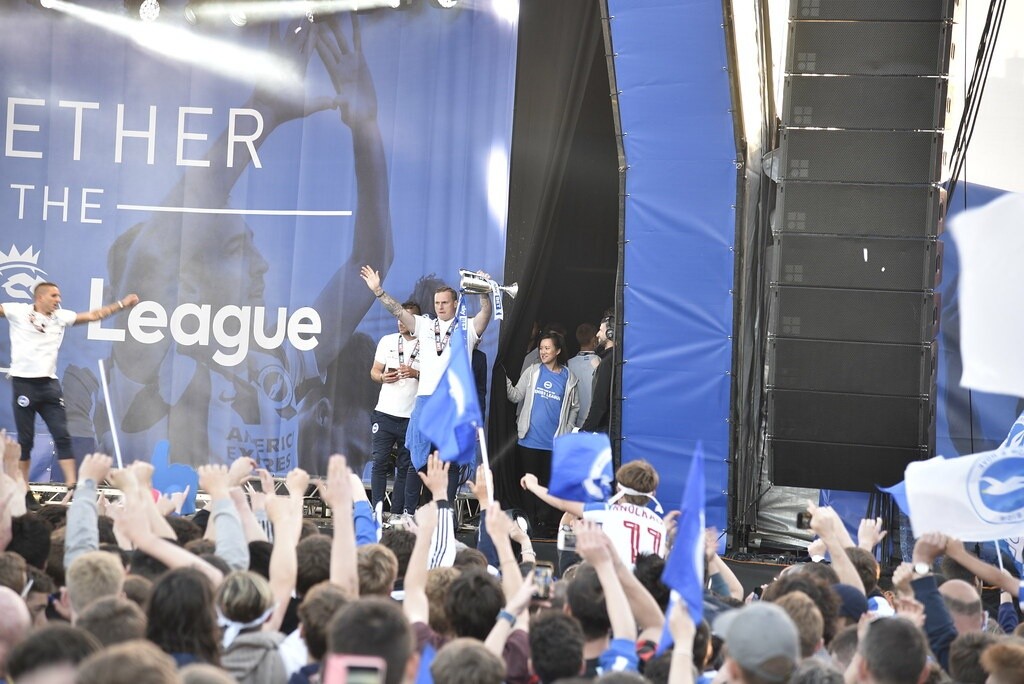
[605,317,614,340]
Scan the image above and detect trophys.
[458,269,519,298]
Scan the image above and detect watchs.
[496,608,517,626]
[912,562,935,574]
[810,555,826,563]
[76,479,97,489]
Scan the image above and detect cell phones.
[321,653,387,684]
[388,368,398,377]
[45,592,62,621]
[530,563,553,600]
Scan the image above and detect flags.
[904,446,1024,543]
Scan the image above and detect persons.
[360,265,492,534]
[60,5,395,486]
[0,284,139,484]
[0,413,1024,684]
[506,307,616,527]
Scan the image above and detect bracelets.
[380,374,383,383]
[416,371,420,380]
[118,301,125,310]
[376,291,385,298]
[520,550,536,556]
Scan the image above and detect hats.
[712,600,800,681]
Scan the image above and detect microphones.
[597,337,610,348]
